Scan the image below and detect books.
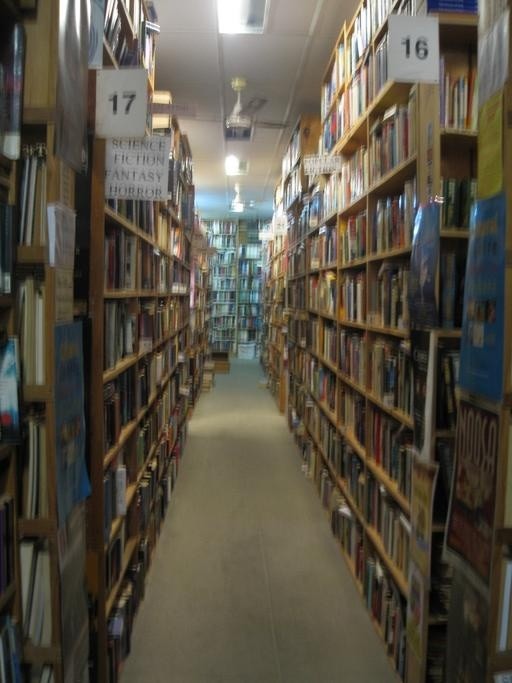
[1,1,216,682]
[200,216,271,352]
[260,0,510,681]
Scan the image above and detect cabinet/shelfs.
[0,0,512,683]
